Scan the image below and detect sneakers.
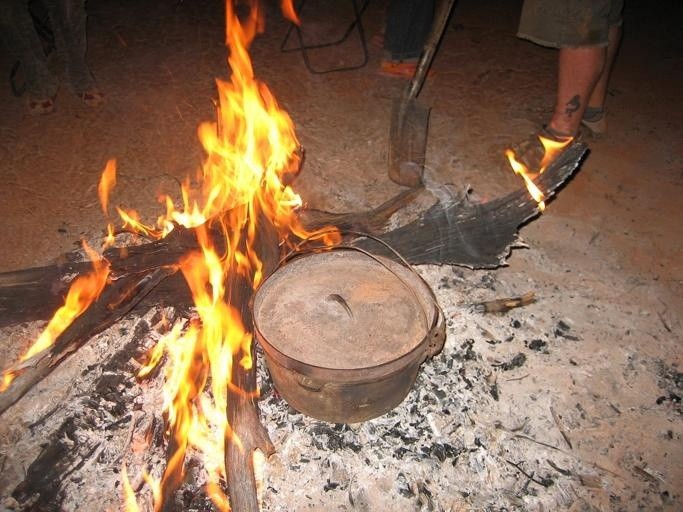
[509,105,607,174]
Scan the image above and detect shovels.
[388,0,455,187]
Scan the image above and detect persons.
[512,0,624,175]
[382,0,437,79]
[0,0,107,115]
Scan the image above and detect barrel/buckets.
[250,228,448,423]
